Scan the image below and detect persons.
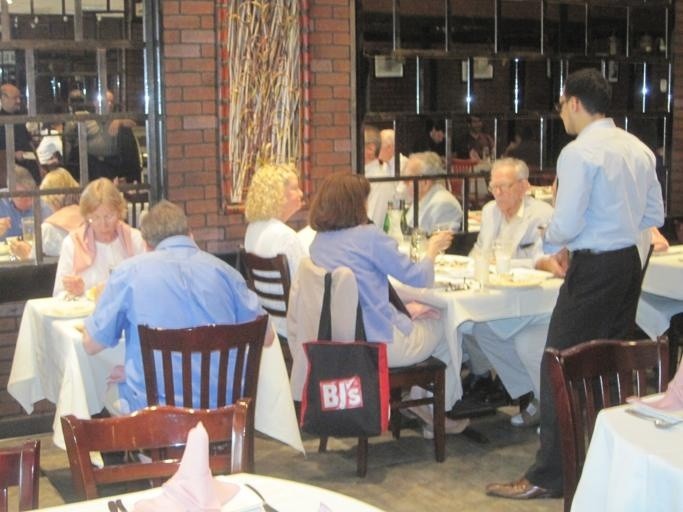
[650,227,669,253]
[487,68,665,498]
[244,165,317,339]
[309,172,470,441]
[52,178,148,300]
[0,83,141,261]
[511,249,573,435]
[364,112,540,237]
[77,200,275,466]
[463,156,554,412]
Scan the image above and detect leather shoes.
[479,376,508,408]
[511,397,541,427]
[485,477,562,500]
[463,373,493,402]
[422,417,470,440]
[406,394,433,426]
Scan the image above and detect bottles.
[409,228,427,263]
[400,200,410,234]
[383,201,394,233]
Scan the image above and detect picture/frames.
[213,0,312,215]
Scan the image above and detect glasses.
[488,179,526,192]
[555,100,576,112]
[3,93,25,99]
[85,212,119,223]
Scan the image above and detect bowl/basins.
[435,253,473,276]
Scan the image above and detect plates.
[490,274,542,288]
[515,268,555,278]
[44,298,96,319]
[440,279,482,295]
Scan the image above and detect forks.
[625,408,683,428]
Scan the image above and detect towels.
[45,203,84,234]
[72,220,133,274]
[625,345,683,411]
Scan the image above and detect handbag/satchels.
[299,341,389,437]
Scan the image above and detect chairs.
[304,259,447,476]
[544,334,670,512]
[442,157,488,210]
[61,398,257,499]
[138,316,270,488]
[0,434,42,512]
[236,245,301,424]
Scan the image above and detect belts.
[574,248,607,257]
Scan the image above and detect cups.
[432,223,450,256]
[21,216,35,243]
